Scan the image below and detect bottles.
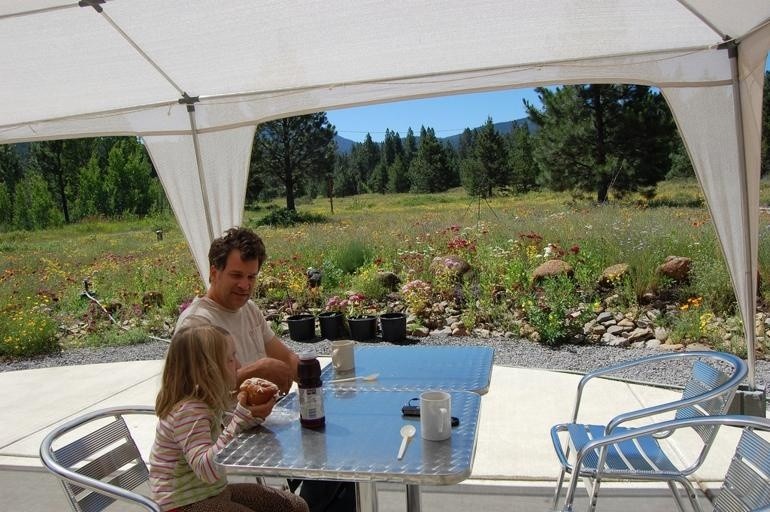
[297,351,325,426]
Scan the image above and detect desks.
[212,344,494,511]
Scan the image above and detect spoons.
[328,373,379,382]
[396,424,416,459]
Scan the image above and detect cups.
[419,391,452,441]
[331,340,355,371]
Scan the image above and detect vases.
[288,312,406,343]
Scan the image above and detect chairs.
[40,406,162,512]
[551,350,768,510]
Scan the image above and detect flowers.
[326,293,370,320]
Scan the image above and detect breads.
[240,377,278,406]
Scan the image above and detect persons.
[178,226,306,396]
[151,322,311,510]
